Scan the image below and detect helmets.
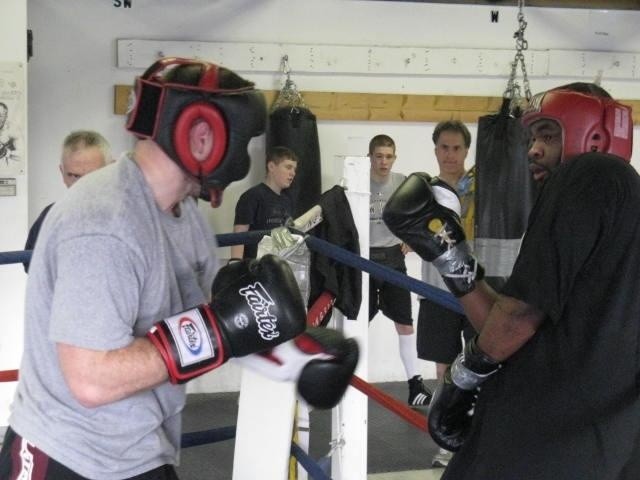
[125,57,267,205]
[521,83,634,165]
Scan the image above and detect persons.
[230,145,300,258]
[0,103,25,158]
[1,57,359,480]
[24,131,116,272]
[381,82,640,480]
[368,135,432,407]
[415,120,476,468]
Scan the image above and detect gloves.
[426,328,503,452]
[146,253,308,386]
[382,171,485,297]
[210,260,359,409]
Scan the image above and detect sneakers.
[430,448,455,467]
[408,375,433,408]
